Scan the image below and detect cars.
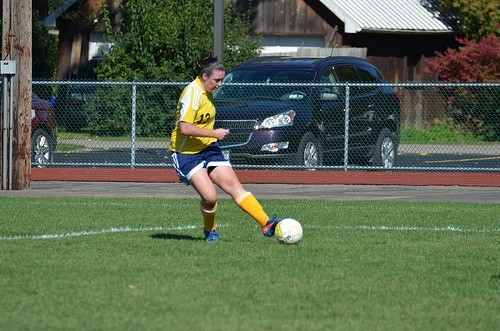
[0,80,58,168]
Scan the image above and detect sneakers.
[204,228,219,241]
[262,219,280,237]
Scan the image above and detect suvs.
[64,56,110,134]
[207,54,402,172]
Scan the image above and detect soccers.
[273,218,303,245]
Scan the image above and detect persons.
[170,53,282,243]
